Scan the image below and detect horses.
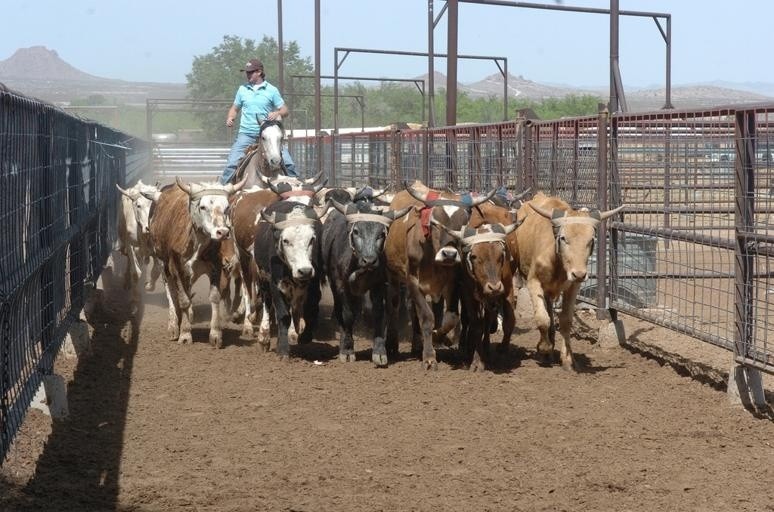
[241,112,288,189]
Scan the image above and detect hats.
[240,59,263,72]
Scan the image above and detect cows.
[114,169,628,377]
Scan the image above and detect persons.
[221,59,300,184]
